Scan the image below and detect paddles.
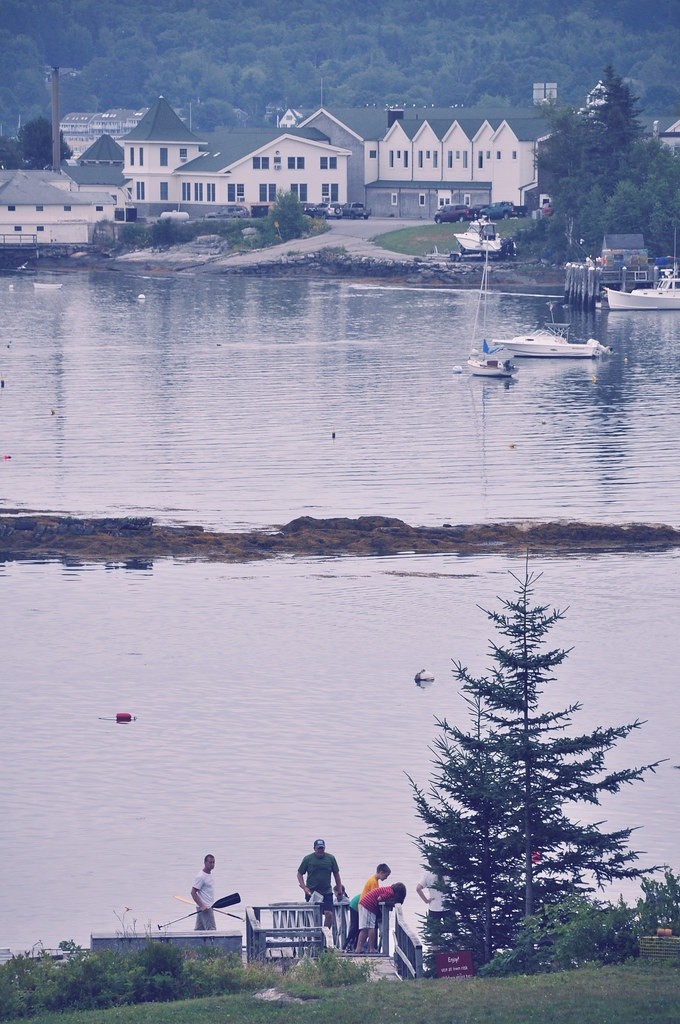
[172,895,246,922]
[157,892,242,931]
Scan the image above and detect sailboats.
[467,235,520,378]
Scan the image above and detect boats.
[31,281,63,289]
[453,216,518,251]
[544,323,571,329]
[602,268,680,309]
[493,326,611,359]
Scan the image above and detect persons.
[355,883,406,954]
[341,863,391,952]
[297,839,351,929]
[416,857,459,951]
[191,855,218,930]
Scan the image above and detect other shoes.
[355,950,366,954]
[341,947,353,952]
[368,949,379,954]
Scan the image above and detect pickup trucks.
[479,201,527,220]
[341,202,371,220]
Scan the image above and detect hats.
[314,839,325,849]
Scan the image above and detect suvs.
[203,205,250,218]
[309,202,343,218]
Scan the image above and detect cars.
[542,201,553,218]
[471,204,489,217]
[434,204,476,224]
[297,202,321,219]
